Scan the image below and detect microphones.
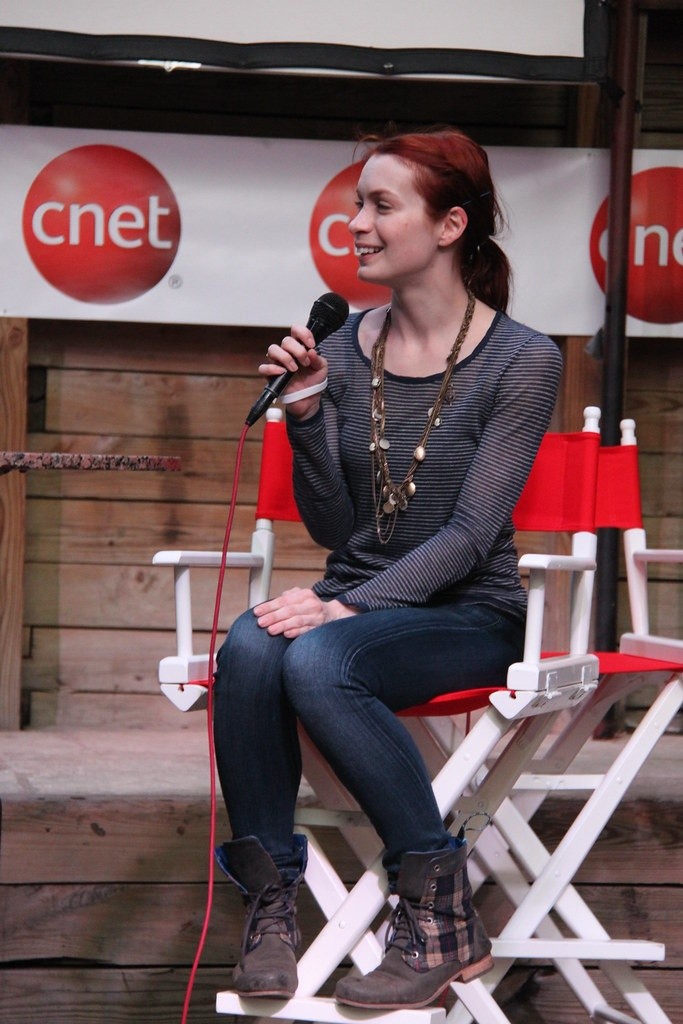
[246,292,350,428]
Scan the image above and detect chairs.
[345,419,683,1024]
[154,403,607,1024]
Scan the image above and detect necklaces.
[368,291,482,548]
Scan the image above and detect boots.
[214,834,308,998]
[336,836,494,1008]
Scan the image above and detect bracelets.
[278,376,330,404]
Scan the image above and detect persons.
[211,124,564,1012]
